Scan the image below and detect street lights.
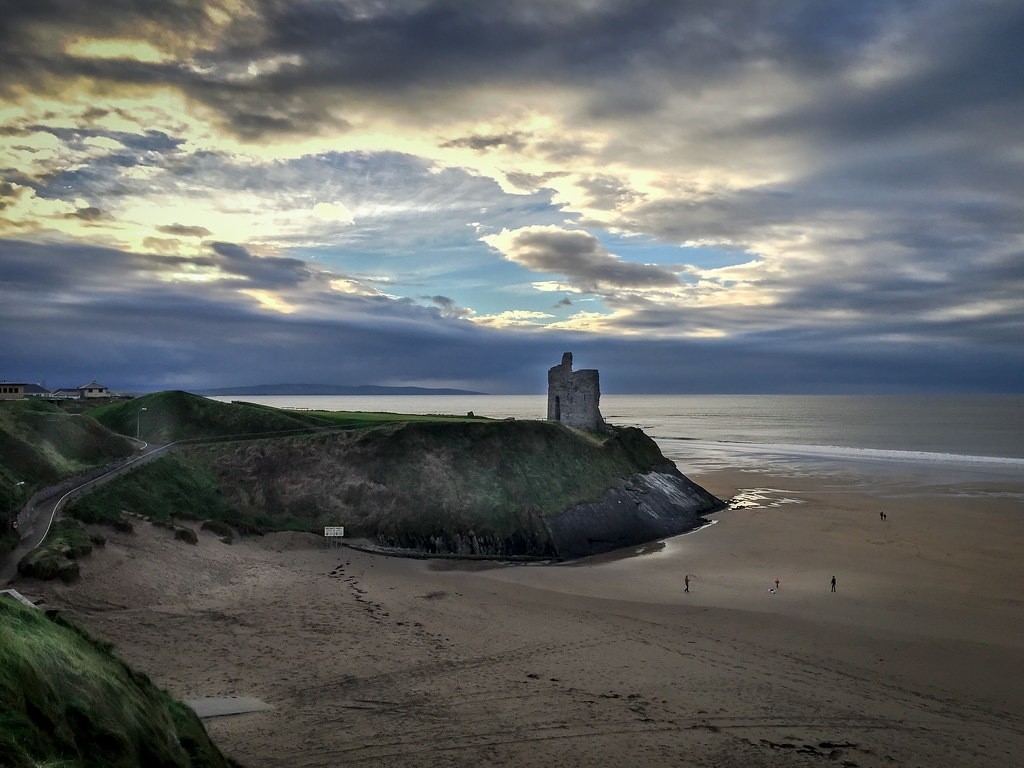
[136,408,148,440]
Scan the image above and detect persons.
[684,574,691,593]
[880,510,888,520]
[831,575,837,592]
[775,578,781,588]
[768,588,775,594]
